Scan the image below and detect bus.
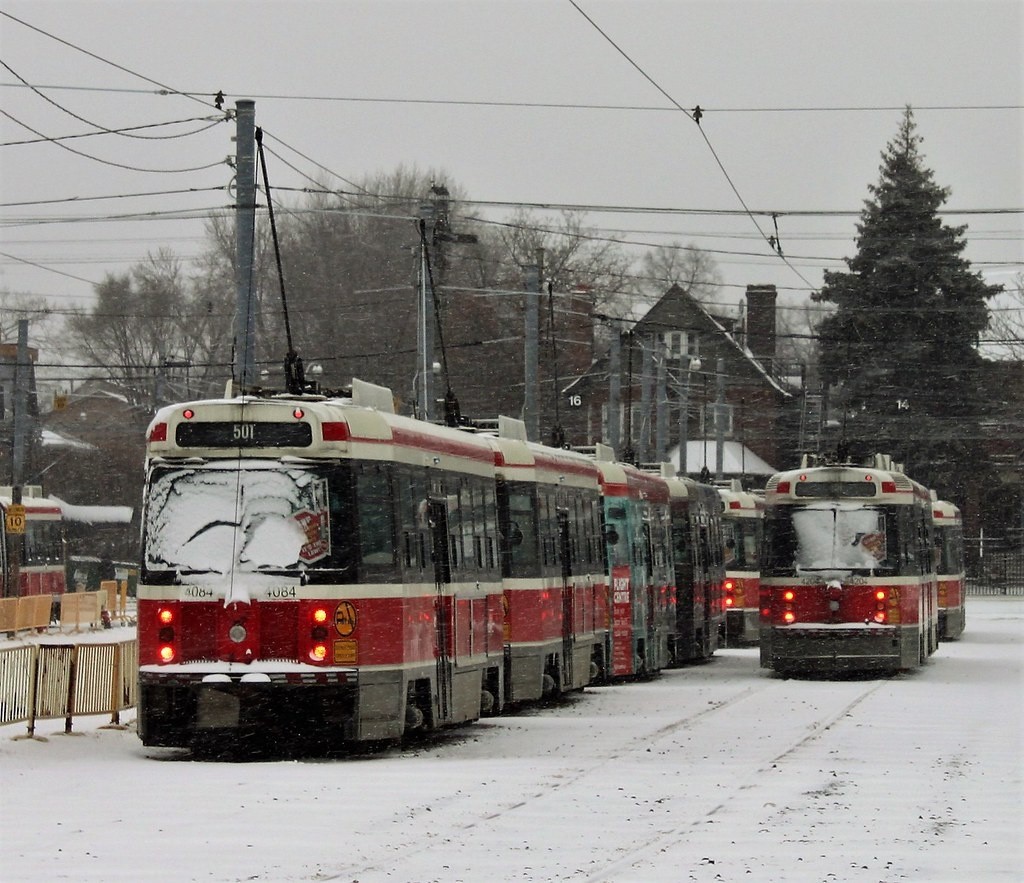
[929,488,969,643]
[713,476,765,648]
[131,377,724,761]
[0,496,136,635]
[757,449,941,680]
